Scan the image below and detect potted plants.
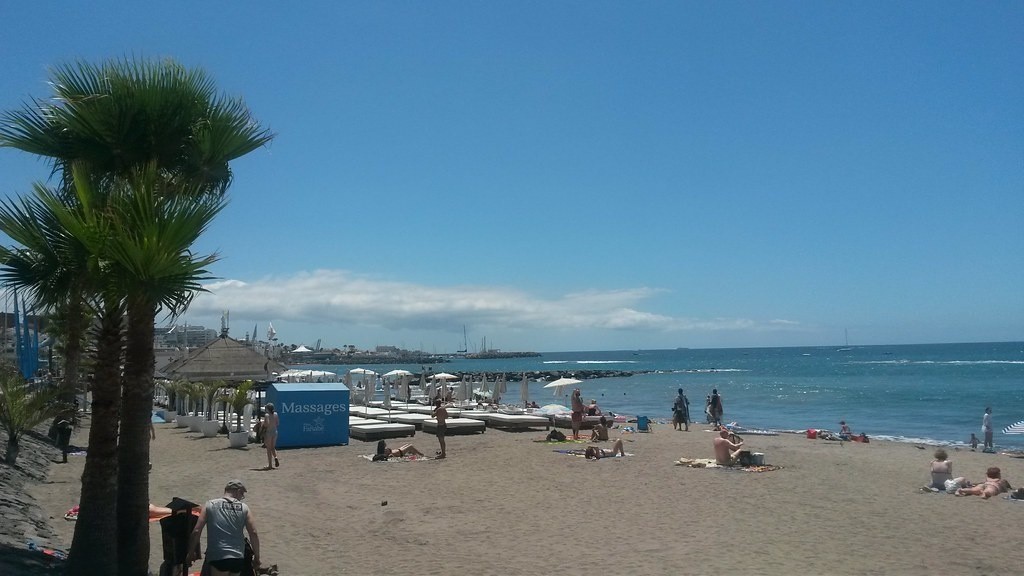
[153,376,255,448]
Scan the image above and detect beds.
[348,399,614,441]
[188,406,266,438]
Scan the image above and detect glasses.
[239,492,245,499]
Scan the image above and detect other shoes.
[148,461,152,470]
[275,457,279,467]
[264,466,272,470]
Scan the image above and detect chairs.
[636,415,653,433]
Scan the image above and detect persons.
[585,438,626,459]
[525,400,539,408]
[930,449,952,489]
[713,430,744,466]
[261,402,279,470]
[838,421,851,434]
[432,399,448,459]
[571,388,584,440]
[185,479,261,576]
[588,398,602,415]
[447,387,455,402]
[590,415,608,441]
[672,388,690,432]
[969,431,981,451]
[705,389,723,430]
[982,406,994,449]
[377,440,424,457]
[955,467,1012,499]
[148,405,156,472]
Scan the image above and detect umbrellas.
[343,367,415,423]
[419,371,529,408]
[473,387,493,401]
[1002,420,1024,435]
[532,403,573,430]
[542,375,583,407]
[276,369,337,382]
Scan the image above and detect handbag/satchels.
[807,429,817,439]
[944,479,962,492]
[859,433,869,442]
